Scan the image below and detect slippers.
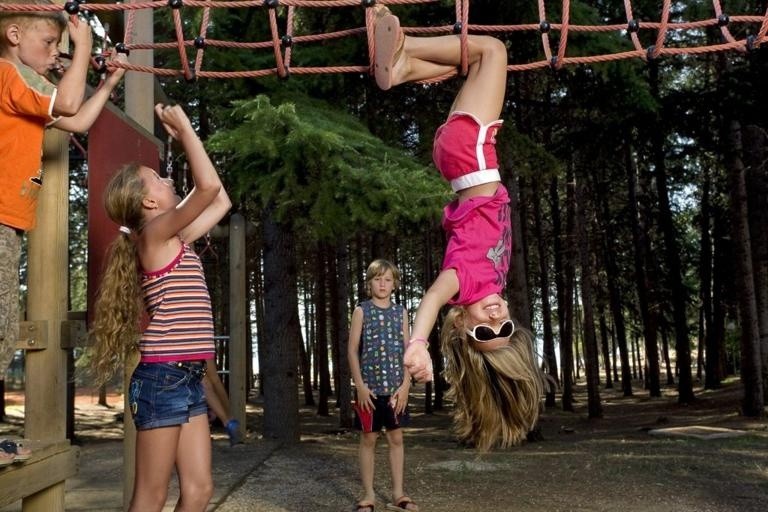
[0,437,33,468]
[372,13,408,92]
[369,4,397,39]
[385,497,418,512]
[355,500,376,512]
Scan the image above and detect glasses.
[460,318,515,342]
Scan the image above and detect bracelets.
[406,337,430,348]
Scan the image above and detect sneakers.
[228,418,243,447]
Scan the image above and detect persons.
[0,0,133,463]
[346,259,422,512]
[370,3,562,463]
[65,103,235,512]
[201,354,242,446]
